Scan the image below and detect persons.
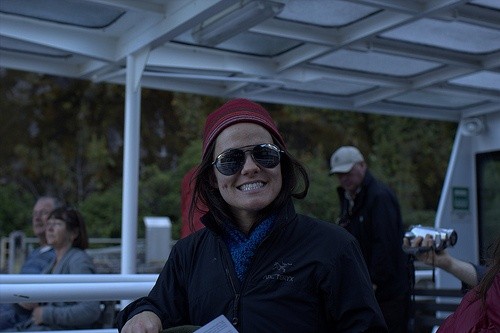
[328,146,417,333]
[0,195,102,333]
[435,238,500,333]
[402,234,489,292]
[117,98,389,333]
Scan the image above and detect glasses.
[211,144,284,177]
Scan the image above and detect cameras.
[405,224,457,252]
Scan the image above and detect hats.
[328,146,365,177]
[202,98,288,152]
